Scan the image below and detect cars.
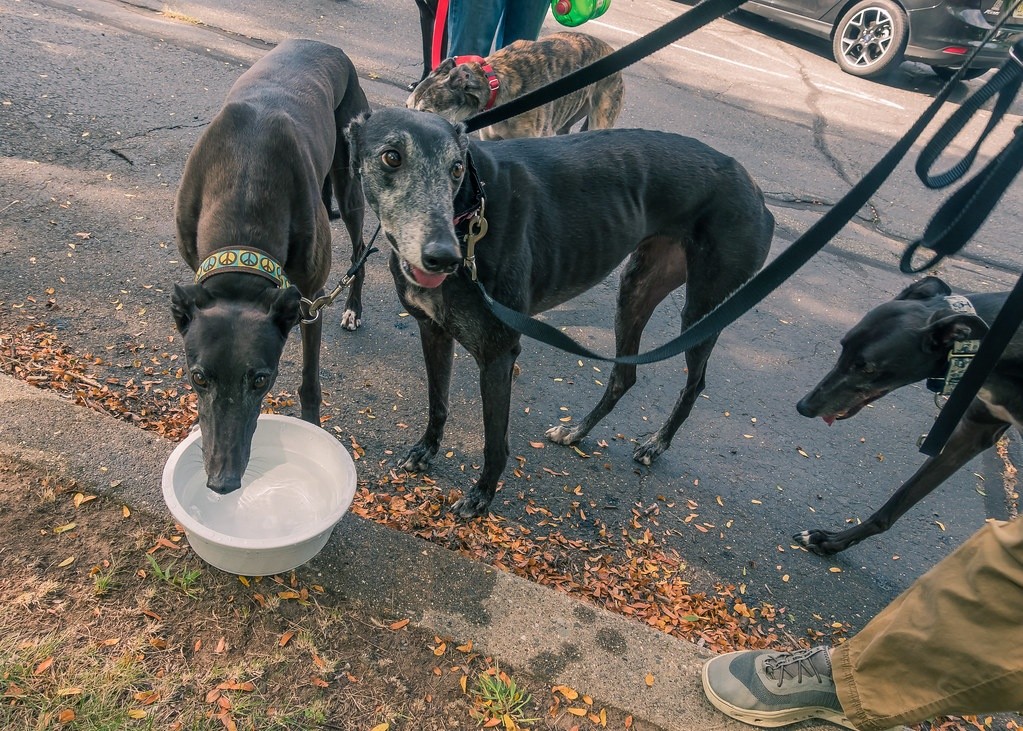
[742,0,1023,82]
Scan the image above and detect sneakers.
[700,646,858,731]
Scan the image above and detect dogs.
[170,38,373,495]
[793,275,1023,555]
[405,30,624,142]
[344,106,776,519]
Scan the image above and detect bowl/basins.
[161,413,357,577]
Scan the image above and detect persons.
[702,513,1023,731]
[448,0,551,58]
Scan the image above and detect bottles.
[551,0,611,27]
